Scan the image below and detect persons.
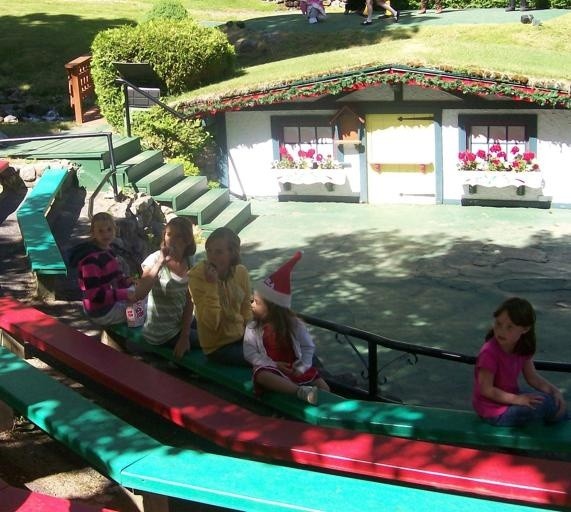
[242,251,331,406]
[505,0,529,12]
[301,0,441,25]
[186,227,254,369]
[71,212,133,327]
[134,216,196,371]
[469,298,571,427]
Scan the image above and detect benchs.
[1,345,164,482]
[316,399,571,461]
[226,416,571,510]
[105,313,346,426]
[120,445,548,511]
[16,169,68,295]
[1,298,258,449]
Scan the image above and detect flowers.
[456,143,538,172]
[272,144,337,168]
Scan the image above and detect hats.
[254,250,304,312]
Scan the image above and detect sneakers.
[295,384,320,406]
